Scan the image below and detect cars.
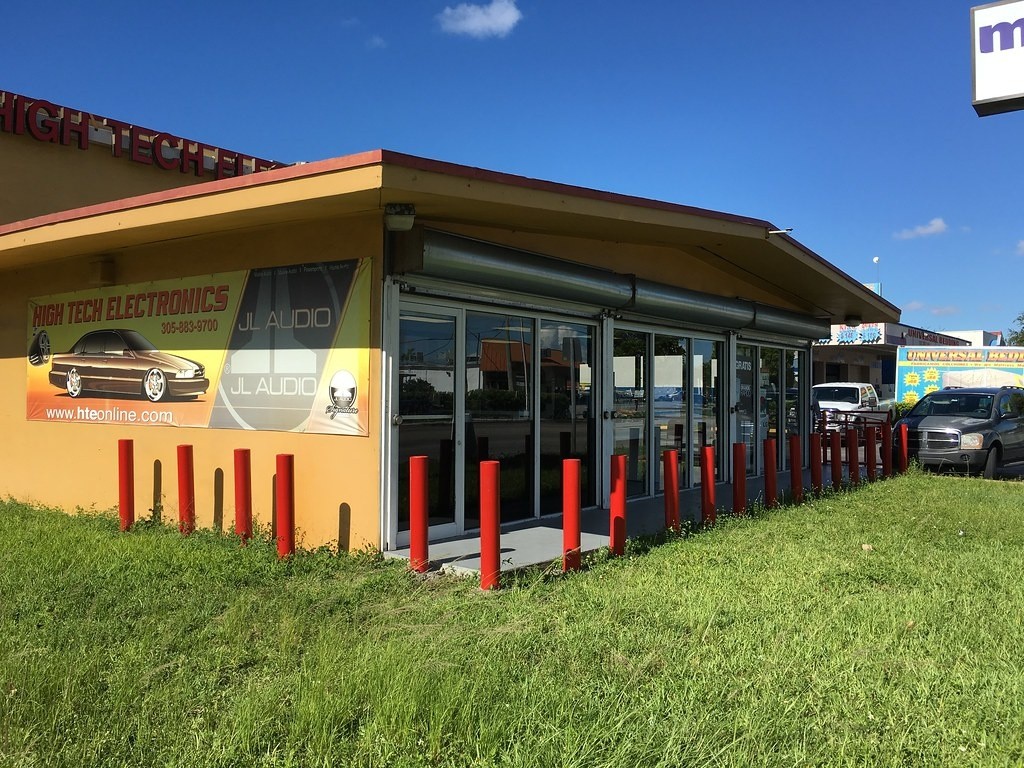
[49,329,212,403]
[668,390,685,402]
[693,395,709,405]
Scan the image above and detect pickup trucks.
[789,382,896,445]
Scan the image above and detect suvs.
[878,385,1024,480]
